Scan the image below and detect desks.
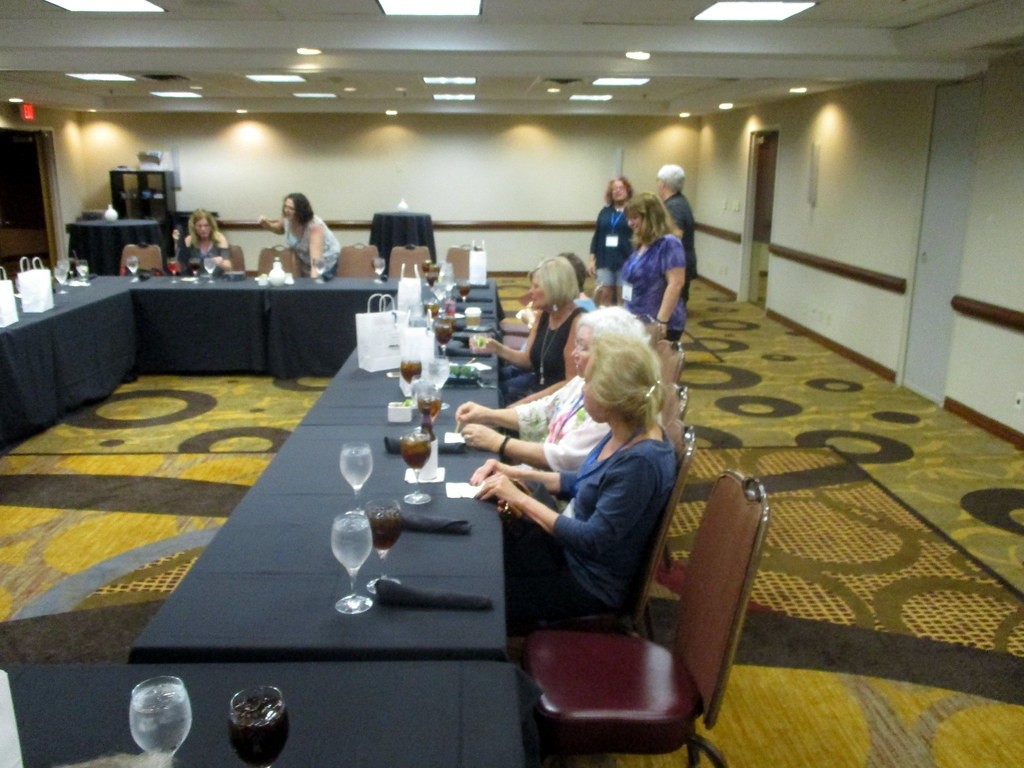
[66,219,163,278]
[0,280,525,768]
[370,213,435,256]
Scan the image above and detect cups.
[422,259,454,332]
[227,685,291,768]
[464,306,481,330]
[129,675,193,757]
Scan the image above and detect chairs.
[387,240,433,277]
[334,245,380,280]
[522,467,770,768]
[258,245,300,276]
[446,244,475,284]
[537,337,695,638]
[119,243,161,277]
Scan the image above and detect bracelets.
[498,434,511,458]
[656,318,669,324]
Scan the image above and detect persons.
[259,193,341,279]
[173,209,232,281]
[589,177,637,307]
[454,250,682,634]
[656,163,698,308]
[623,192,687,345]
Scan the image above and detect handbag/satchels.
[0,266,20,329]
[468,241,488,288]
[356,294,408,372]
[397,261,424,321]
[18,255,54,315]
[400,309,436,397]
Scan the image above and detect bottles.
[104,204,118,222]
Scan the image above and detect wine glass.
[126,256,140,283]
[425,355,450,410]
[416,385,442,425]
[400,355,423,397]
[365,498,402,594]
[400,429,432,505]
[339,441,374,511]
[166,257,179,284]
[434,322,453,356]
[371,258,386,284]
[458,282,470,301]
[330,511,374,615]
[189,257,202,284]
[53,258,89,295]
[203,258,217,284]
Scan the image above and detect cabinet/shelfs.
[110,164,177,254]
